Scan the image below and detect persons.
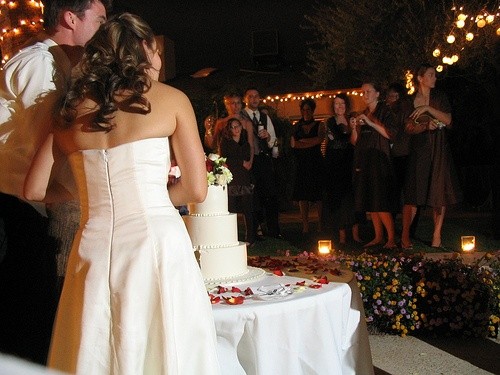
[204,65,457,250]
[0,0,216,375]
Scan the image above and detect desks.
[208,257,375,375]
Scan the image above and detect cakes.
[180,154,248,281]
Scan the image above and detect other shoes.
[272,232,287,240]
[302,226,311,238]
[353,237,364,244]
[339,237,348,247]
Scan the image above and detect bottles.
[272,141,279,158]
[258,121,264,138]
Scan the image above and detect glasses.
[334,102,345,106]
[230,122,242,130]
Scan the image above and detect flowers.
[277,248,500,341]
[204,151,234,187]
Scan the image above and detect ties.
[252,113,258,125]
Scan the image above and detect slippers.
[401,243,414,250]
[364,241,398,251]
[431,244,447,249]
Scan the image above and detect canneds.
[258,125,264,136]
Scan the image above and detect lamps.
[318,239,333,254]
[461,235,476,252]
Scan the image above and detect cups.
[205,116,216,136]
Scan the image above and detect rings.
[415,112,418,114]
